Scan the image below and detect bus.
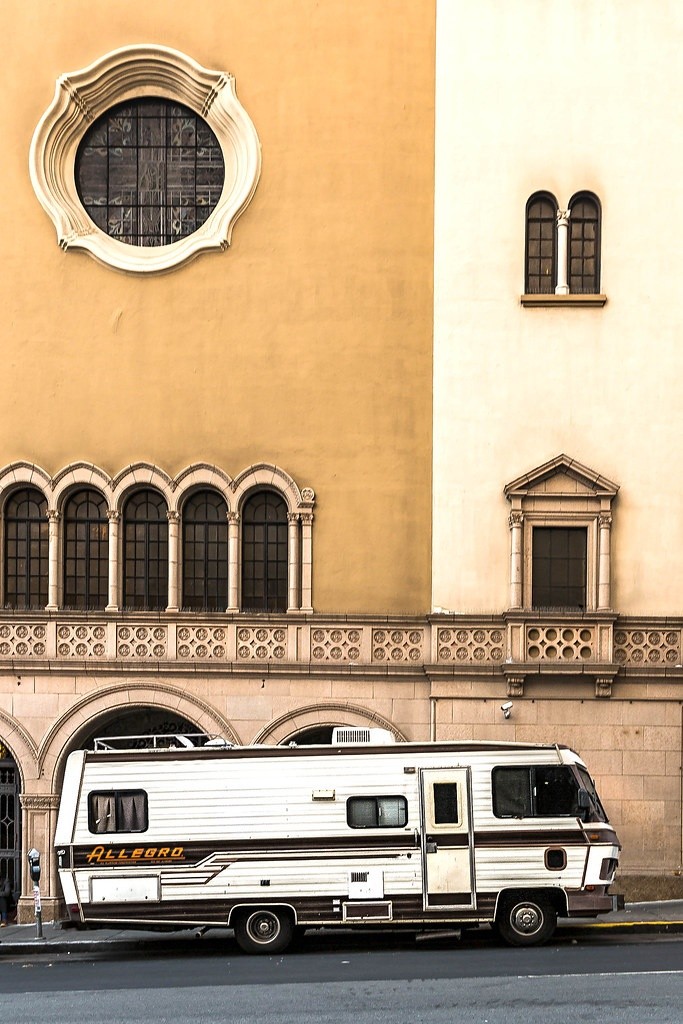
[54,729,627,958]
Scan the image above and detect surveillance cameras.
[501,701,513,711]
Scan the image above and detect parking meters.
[26,847,46,940]
